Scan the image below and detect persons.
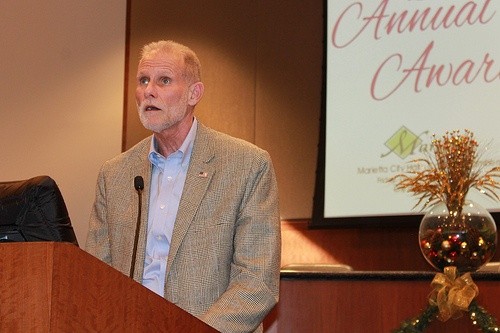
[85,40,281,332]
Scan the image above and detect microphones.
[129,176,145,280]
